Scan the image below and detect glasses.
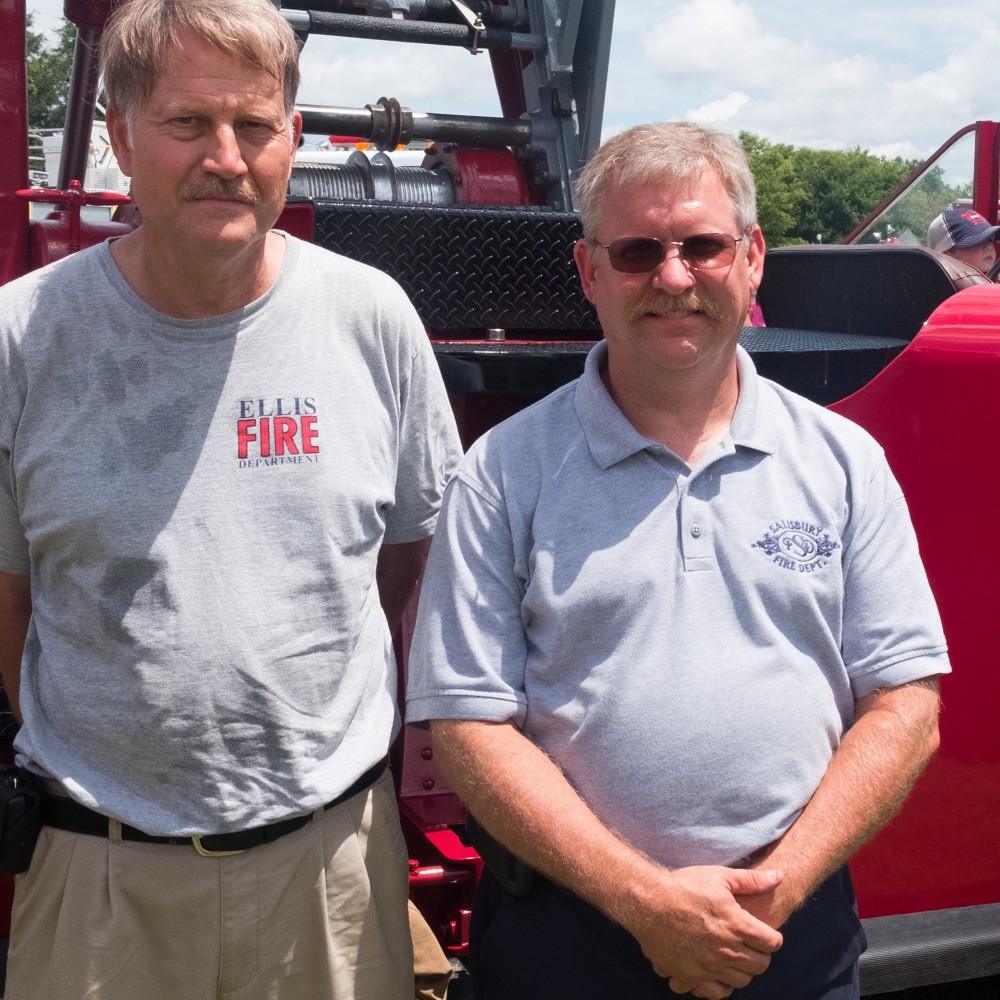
[591,232,743,274]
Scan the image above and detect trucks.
[0,0,1000,997]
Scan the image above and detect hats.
[927,207,1000,252]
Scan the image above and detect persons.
[0,0,466,1000]
[927,207,1000,273]
[406,120,952,1000]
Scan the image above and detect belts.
[43,751,390,855]
